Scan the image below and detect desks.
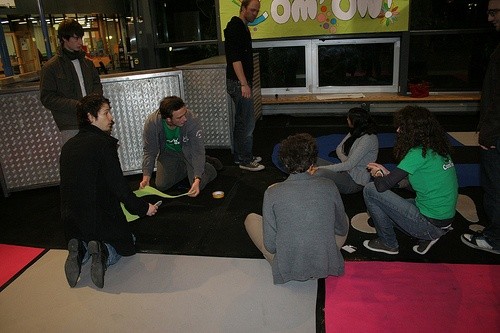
[0,55,236,198]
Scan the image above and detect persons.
[461,1,500,254]
[244,135,349,284]
[60,94,158,288]
[40,19,102,145]
[140,96,223,197]
[364,106,458,254]
[225,1,264,172]
[307,107,379,193]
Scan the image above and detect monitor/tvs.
[252,39,312,96]
[399,29,500,96]
[311,37,400,93]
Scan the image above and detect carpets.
[0,249,318,333]
[325,261,500,333]
[0,244,45,287]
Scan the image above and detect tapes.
[212,191,225,199]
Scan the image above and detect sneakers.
[363,239,399,254]
[234,156,262,162]
[239,162,265,171]
[460,233,500,254]
[413,238,440,254]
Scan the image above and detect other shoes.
[63,238,87,288]
[205,155,223,170]
[88,241,110,289]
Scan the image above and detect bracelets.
[192,176,202,183]
[240,83,249,88]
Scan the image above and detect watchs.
[374,169,384,177]
